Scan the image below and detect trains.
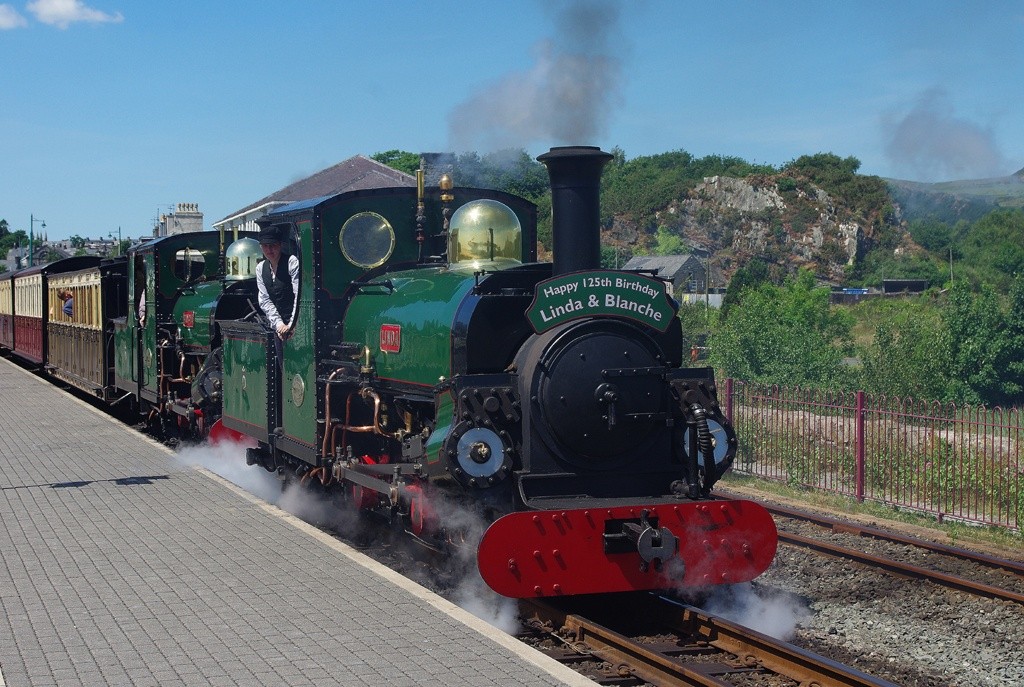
[0,143,780,599]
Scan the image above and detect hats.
[258,226,284,244]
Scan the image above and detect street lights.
[107,225,121,257]
[29,212,47,267]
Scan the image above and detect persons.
[688,344,696,366]
[139,288,145,327]
[57,288,73,318]
[255,226,299,374]
